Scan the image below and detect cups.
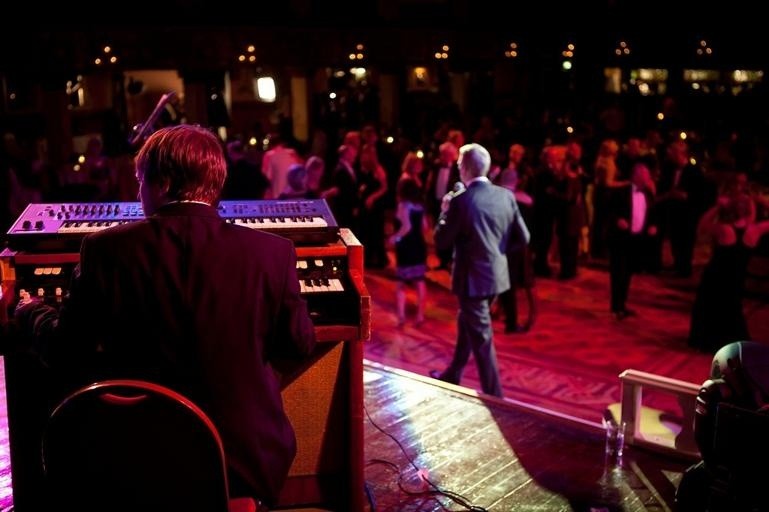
[601,452,624,492]
[602,416,628,456]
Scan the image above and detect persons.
[9,123,316,502]
[1,108,469,324]
[428,139,534,401]
[489,73,769,357]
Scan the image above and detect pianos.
[8,257,359,325]
[6,198,341,253]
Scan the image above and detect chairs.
[39,379,257,512]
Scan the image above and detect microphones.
[454,182,464,193]
